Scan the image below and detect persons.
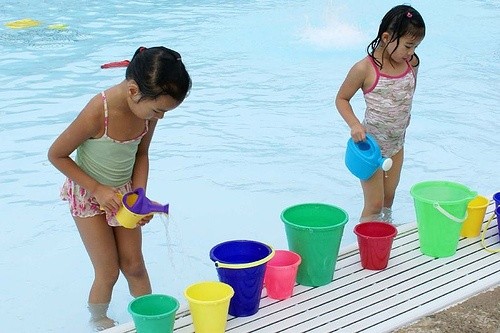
[46,46,192,304]
[335,5,426,217]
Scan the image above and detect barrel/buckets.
[209,239,276,317]
[461,196,489,238]
[183,281,234,333]
[264,250,302,300]
[354,221,398,271]
[492,192,500,207]
[410,180,471,253]
[482,206,500,252]
[127,293,179,333]
[280,203,348,287]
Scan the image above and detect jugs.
[99,187,169,226]
[344,132,393,180]
